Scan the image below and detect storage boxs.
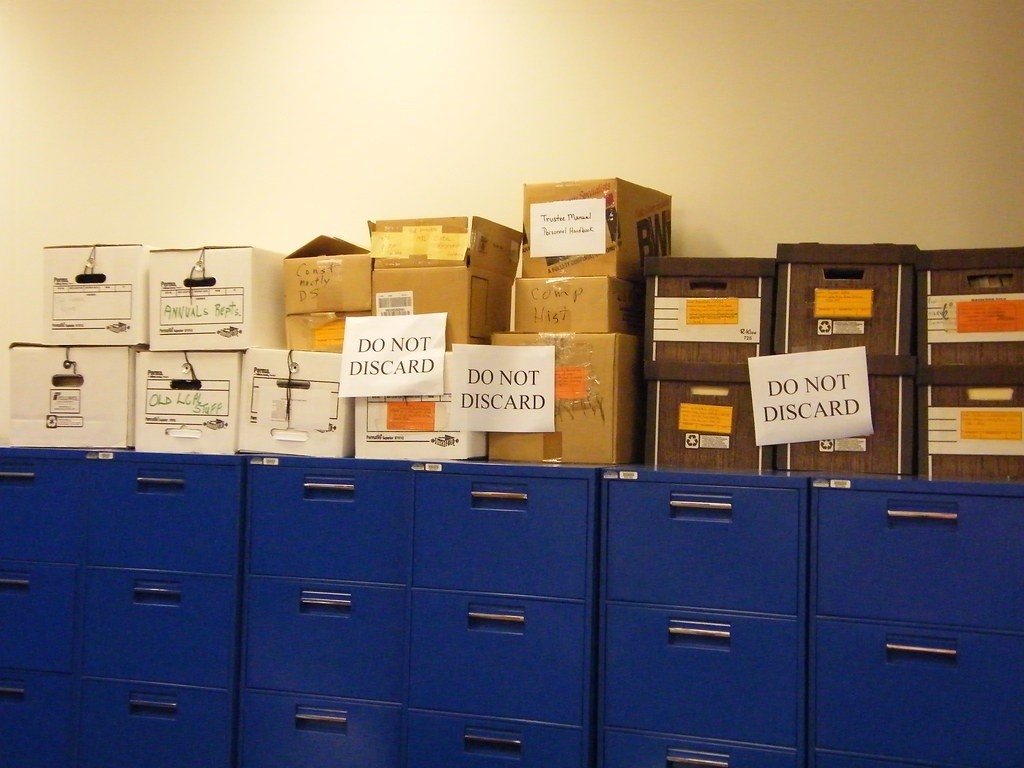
[8,178,1024,482]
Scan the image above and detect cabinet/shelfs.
[1,447,1024,768]
